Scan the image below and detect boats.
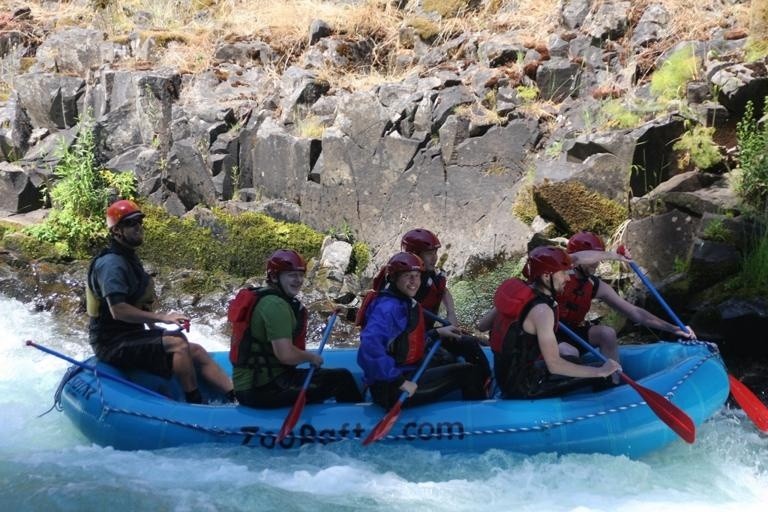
[54,341,729,461]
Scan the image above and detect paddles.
[616,246,768,433]
[276,310,339,443]
[423,307,489,348]
[362,339,442,446]
[25,340,169,401]
[559,321,695,445]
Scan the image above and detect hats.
[124,212,146,221]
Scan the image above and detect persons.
[401,229,455,327]
[561,233,697,383]
[477,246,620,398]
[354,252,488,408]
[235,248,363,408]
[86,201,233,405]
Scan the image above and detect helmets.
[527,246,574,276]
[401,229,441,253]
[106,200,141,228]
[385,253,425,279]
[266,249,307,275]
[567,232,606,254]
